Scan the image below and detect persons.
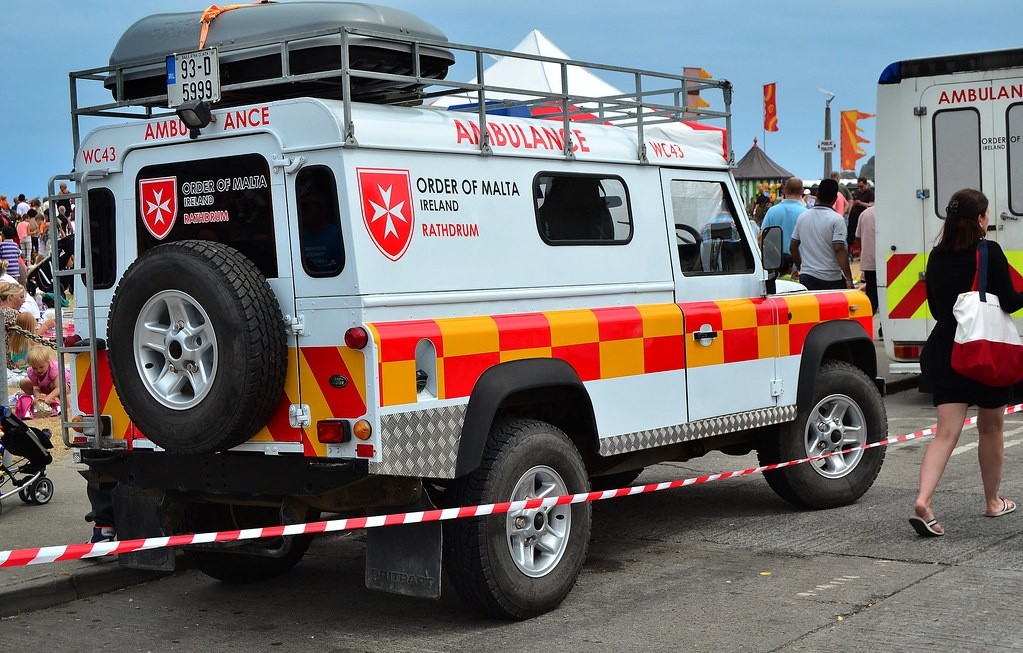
[909,189,1023,538]
[0,182,75,376]
[19,345,70,412]
[748,171,883,340]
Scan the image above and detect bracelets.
[42,323,51,331]
[34,231,36,234]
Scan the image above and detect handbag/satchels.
[951,238,1023,386]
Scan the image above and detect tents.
[429,30,730,169]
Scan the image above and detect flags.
[764,83,778,131]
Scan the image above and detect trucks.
[878,47,1023,392]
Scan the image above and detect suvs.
[49,1,888,620]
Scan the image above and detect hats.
[803,188,811,194]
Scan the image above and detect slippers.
[984,497,1016,517]
[909,516,944,537]
